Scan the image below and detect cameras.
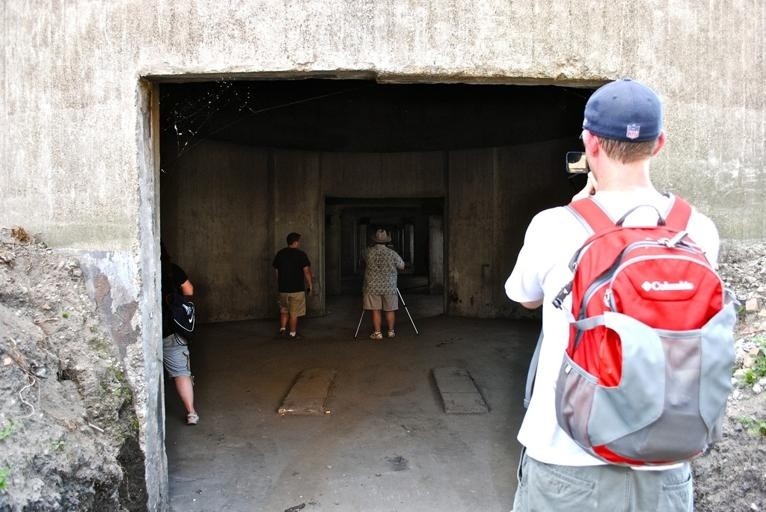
[564,151,592,176]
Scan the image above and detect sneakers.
[186,411,200,425]
[369,329,396,340]
[278,328,297,340]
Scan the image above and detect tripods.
[354,287,420,341]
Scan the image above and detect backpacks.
[550,190,737,471]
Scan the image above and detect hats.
[578,78,663,142]
[371,228,392,243]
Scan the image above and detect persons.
[498,78,739,511]
[273,232,313,337]
[160,246,199,425]
[363,230,405,340]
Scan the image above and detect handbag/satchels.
[171,299,196,335]
